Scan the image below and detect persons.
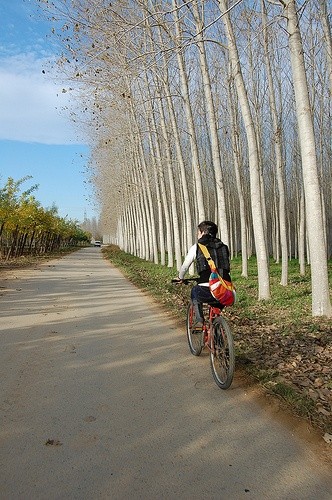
[172,221,232,353]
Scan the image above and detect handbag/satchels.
[209,271,237,305]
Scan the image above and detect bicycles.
[171,278,236,390]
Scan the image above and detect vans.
[94,241,101,247]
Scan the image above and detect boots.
[213,318,225,344]
[192,299,205,327]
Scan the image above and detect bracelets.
[177,276,183,280]
